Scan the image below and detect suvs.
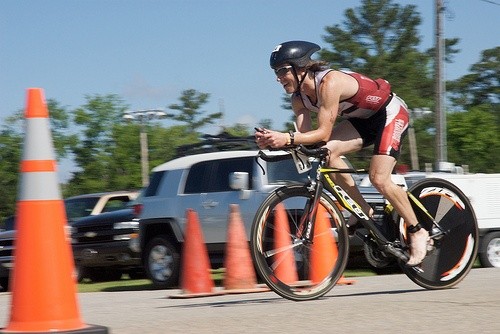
[131,147,410,290]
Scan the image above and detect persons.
[254,40,430,267]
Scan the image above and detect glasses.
[274,67,292,78]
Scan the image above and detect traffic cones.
[0,86,113,334]
[218,204,271,293]
[167,209,223,299]
[262,200,316,292]
[301,199,355,287]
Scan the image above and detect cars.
[68,186,146,282]
[0,190,140,292]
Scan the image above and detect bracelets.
[289,130,295,147]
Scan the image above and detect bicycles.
[249,140,479,302]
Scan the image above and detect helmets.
[270,41,321,68]
